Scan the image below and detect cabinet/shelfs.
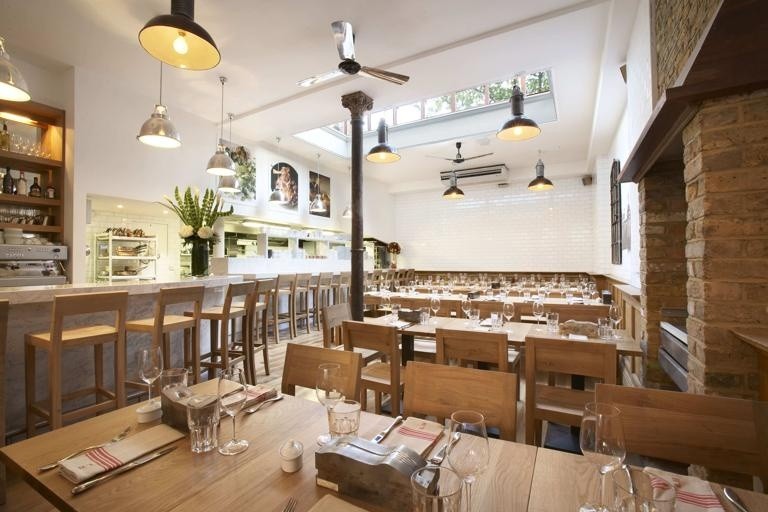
[92,229,158,284]
[0,94,67,282]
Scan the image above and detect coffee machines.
[0,242,69,287]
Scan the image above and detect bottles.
[0,119,10,152]
[0,166,41,197]
[279,437,303,475]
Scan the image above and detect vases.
[389,253,397,270]
[190,236,210,278]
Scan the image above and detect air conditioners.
[438,162,510,187]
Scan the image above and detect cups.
[327,398,361,440]
[0,205,43,225]
[185,394,219,454]
[409,466,463,512]
[47,185,56,199]
[10,133,51,159]
[159,365,191,396]
[569,398,679,512]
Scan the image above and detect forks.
[36,423,134,474]
[426,432,461,466]
[282,497,299,512]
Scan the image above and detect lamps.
[134,60,353,220]
[440,168,465,200]
[0,31,31,104]
[365,117,402,163]
[136,0,222,72]
[527,158,554,191]
[493,74,542,141]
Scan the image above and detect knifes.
[370,415,403,444]
[199,386,244,410]
[68,446,181,496]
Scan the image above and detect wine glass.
[314,361,343,448]
[136,345,164,412]
[216,368,252,457]
[368,272,624,342]
[447,408,491,512]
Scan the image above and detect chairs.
[281,320,767,491]
[273,270,350,347]
[2,299,9,364]
[183,273,271,386]
[364,269,414,288]
[25,290,128,438]
[125,285,206,402]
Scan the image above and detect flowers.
[387,241,401,255]
[151,180,237,247]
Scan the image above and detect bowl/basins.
[99,270,109,276]
[0,227,48,246]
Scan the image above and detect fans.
[423,141,493,167]
[295,20,411,90]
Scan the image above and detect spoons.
[242,393,287,414]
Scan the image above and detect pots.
[115,265,150,276]
[116,244,147,256]
